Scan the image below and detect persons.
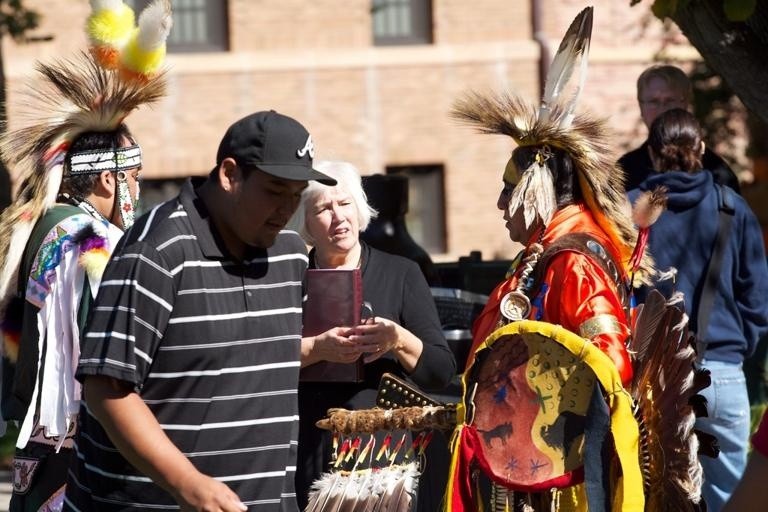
[624,109,768,512]
[289,160,457,511]
[459,144,634,512]
[61,109,340,511]
[613,65,740,196]
[0,126,142,512]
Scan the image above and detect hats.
[217,111,337,187]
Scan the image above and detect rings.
[376,343,384,353]
